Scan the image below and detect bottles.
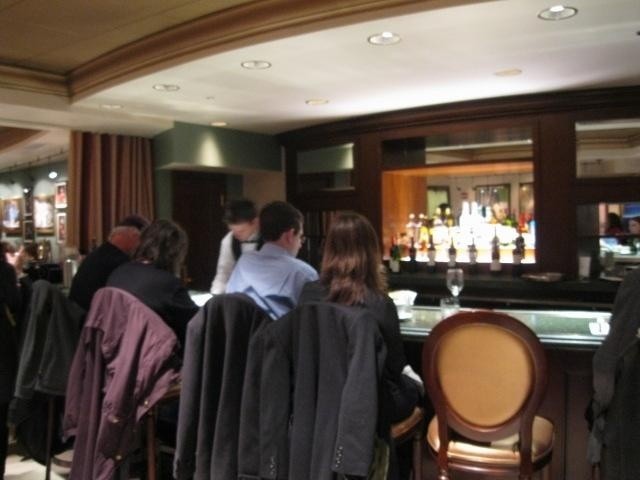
[405,206,455,250]
[512,225,526,280]
[388,235,400,273]
[495,208,518,246]
[467,236,480,276]
[445,237,457,268]
[425,234,437,274]
[408,235,418,274]
[490,226,503,277]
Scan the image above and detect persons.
[1,244,26,267]
[592,268,640,479]
[2,201,22,229]
[626,215,639,253]
[228,199,321,325]
[293,208,426,477]
[604,212,626,245]
[106,218,201,479]
[57,184,67,205]
[65,214,152,319]
[492,201,519,230]
[211,199,262,298]
[58,217,65,242]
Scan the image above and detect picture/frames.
[0,181,68,244]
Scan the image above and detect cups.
[439,268,465,321]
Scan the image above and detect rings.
[14,251,21,258]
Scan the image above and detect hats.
[117,213,151,232]
